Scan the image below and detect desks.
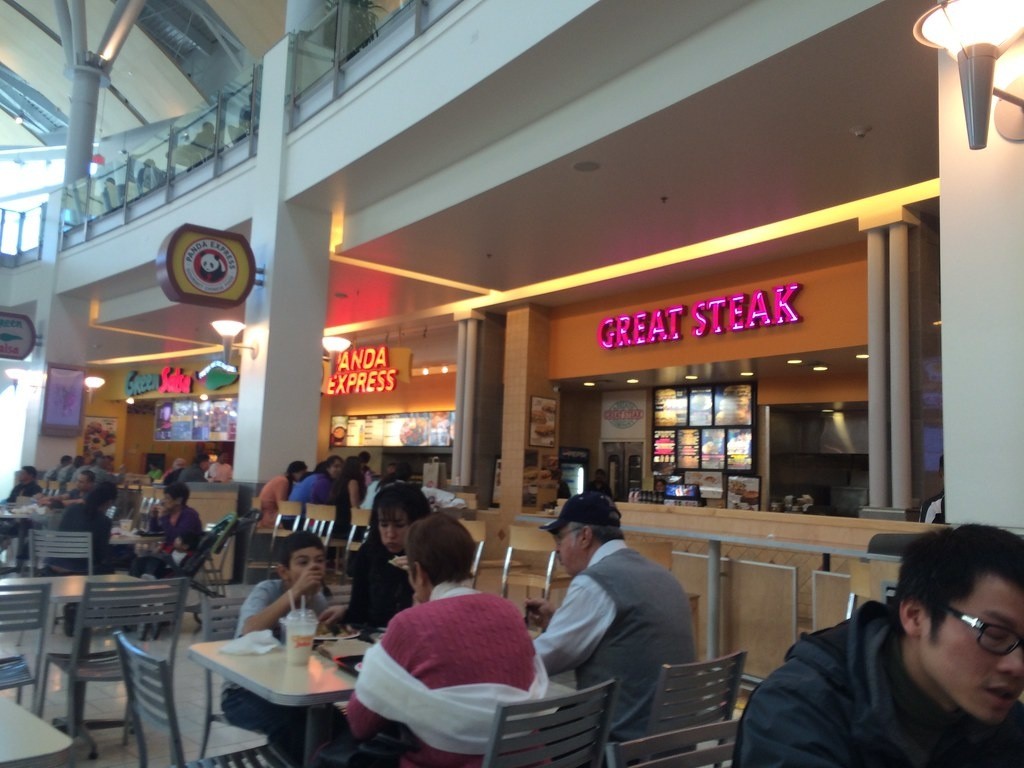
[0,696,74,768]
[188,632,385,768]
[109,535,166,544]
[0,574,171,759]
[0,512,32,574]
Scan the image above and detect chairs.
[483,650,747,768]
[0,511,301,768]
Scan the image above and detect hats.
[345,456,360,463]
[174,459,186,469]
[538,491,621,531]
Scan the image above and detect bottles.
[640,489,665,504]
[359,425,364,444]
[629,489,640,502]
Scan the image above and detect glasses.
[936,604,1024,655]
[553,527,582,547]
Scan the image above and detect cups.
[120,520,132,533]
[279,589,317,666]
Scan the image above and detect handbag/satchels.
[423,457,448,491]
[315,721,421,768]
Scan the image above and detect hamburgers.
[531,401,556,437]
[654,388,677,427]
[716,386,754,426]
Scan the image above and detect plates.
[313,630,361,640]
[379,628,387,632]
[355,663,362,672]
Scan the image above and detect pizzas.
[388,554,409,569]
[315,623,351,638]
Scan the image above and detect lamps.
[912,0,1024,150]
[211,320,260,367]
[85,377,105,405]
[322,336,351,374]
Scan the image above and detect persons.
[588,469,613,499]
[7,452,232,507]
[733,524,1024,768]
[349,514,549,768]
[346,482,432,628]
[532,493,696,768]
[163,532,196,578]
[103,91,254,213]
[221,531,351,768]
[43,481,117,575]
[257,450,414,530]
[130,483,203,579]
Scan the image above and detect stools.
[119,485,164,514]
[37,480,76,497]
[846,560,901,624]
[625,540,699,651]
[459,518,574,633]
[243,498,374,585]
